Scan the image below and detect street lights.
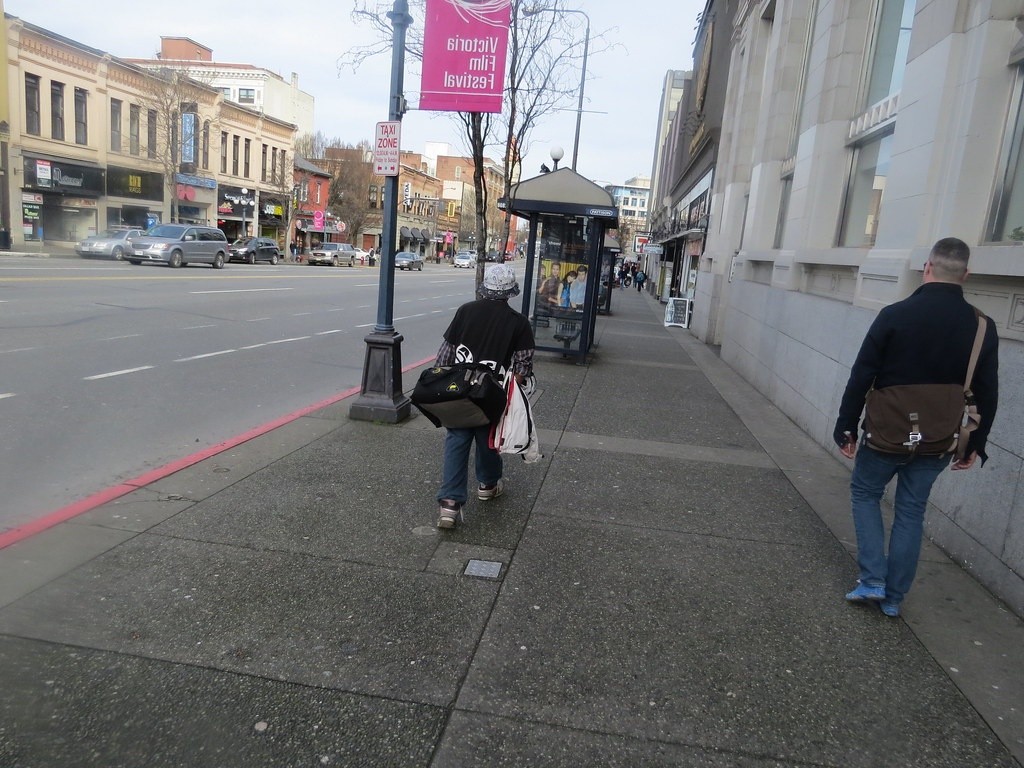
[522,7,589,171]
[234,188,256,236]
[469,232,475,249]
[494,235,501,250]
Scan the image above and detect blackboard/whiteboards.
[664,298,690,326]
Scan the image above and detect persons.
[833,237,999,616]
[435,263,535,529]
[538,262,587,309]
[369,246,373,255]
[618,263,647,292]
[290,240,297,252]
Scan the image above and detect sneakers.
[436,497,464,530]
[478,478,505,500]
[845,585,885,603]
[881,600,899,616]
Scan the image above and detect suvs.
[123,223,231,269]
[308,242,356,268]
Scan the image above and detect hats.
[478,264,520,299]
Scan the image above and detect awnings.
[401,228,433,242]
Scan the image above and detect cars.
[74,228,145,261]
[394,252,424,271]
[453,250,515,268]
[229,236,280,265]
[354,247,369,261]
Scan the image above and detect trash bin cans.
[369,258,375,266]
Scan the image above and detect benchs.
[552,320,582,358]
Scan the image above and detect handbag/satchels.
[862,383,967,458]
[410,363,506,430]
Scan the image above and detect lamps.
[691,12,704,45]
[697,217,707,235]
[679,221,687,232]
